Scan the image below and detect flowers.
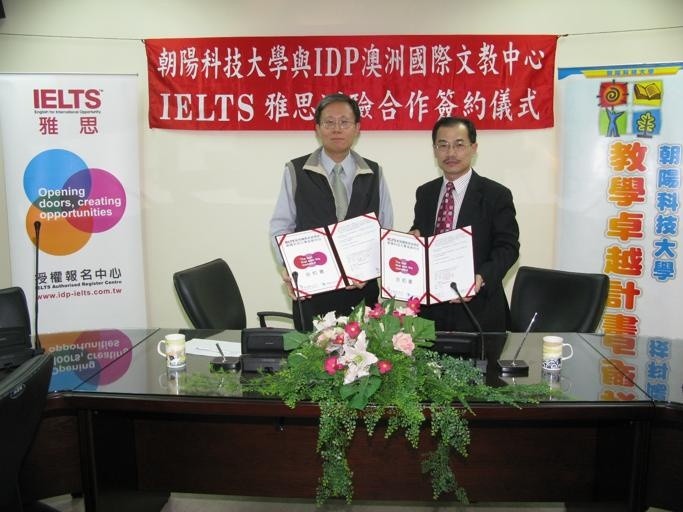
[180,295,573,506]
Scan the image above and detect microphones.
[450,282,488,374]
[34,221,44,355]
[292,271,305,331]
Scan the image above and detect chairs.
[0,353,56,511]
[173,259,295,330]
[510,266,610,332]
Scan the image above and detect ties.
[435,182,455,233]
[331,165,348,222]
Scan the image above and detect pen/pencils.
[511,313,537,364]
[216,343,226,360]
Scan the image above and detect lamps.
[0,287,31,336]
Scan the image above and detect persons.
[401,118,522,332]
[269,95,396,335]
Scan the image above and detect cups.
[159,366,189,397]
[157,333,187,369]
[538,371,573,401]
[540,335,574,370]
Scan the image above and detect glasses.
[320,117,355,130]
[433,142,473,152]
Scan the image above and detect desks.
[0,328,159,512]
[579,333,683,512]
[75,328,652,512]
